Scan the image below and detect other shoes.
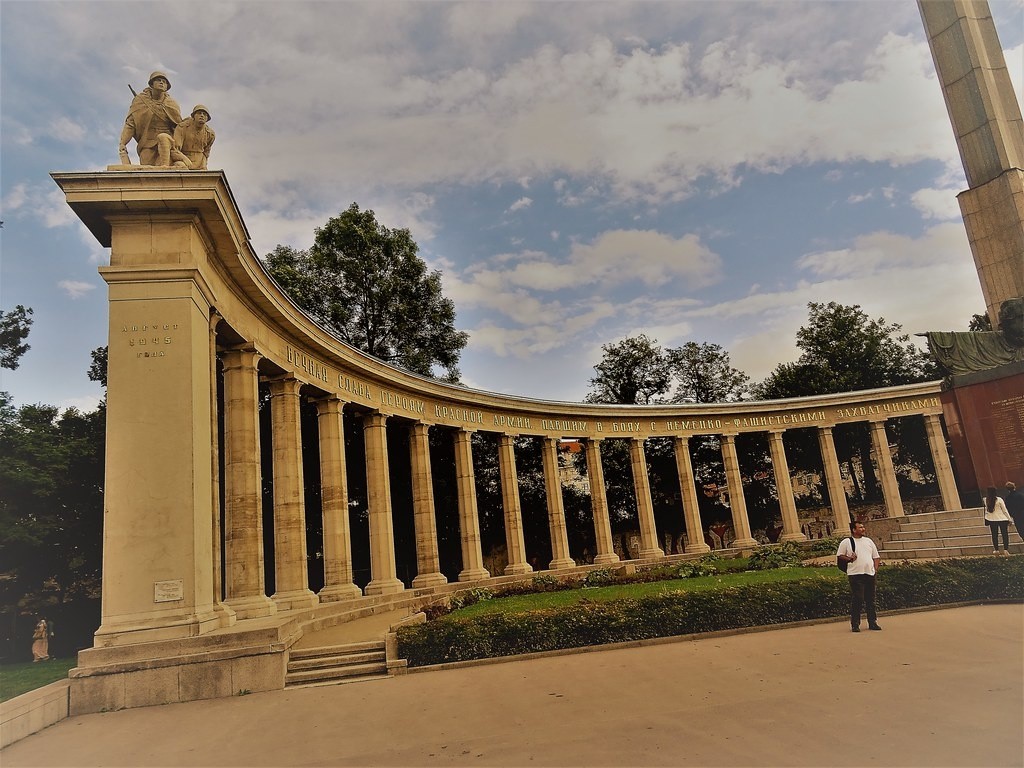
[1004,550,1009,557]
[992,551,1000,557]
[852,622,860,632]
[869,621,881,630]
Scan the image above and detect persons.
[1004,481,1024,540]
[837,521,883,632]
[119,71,216,170]
[983,487,1013,555]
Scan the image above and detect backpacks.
[838,537,855,573]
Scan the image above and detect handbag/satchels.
[984,519,989,526]
